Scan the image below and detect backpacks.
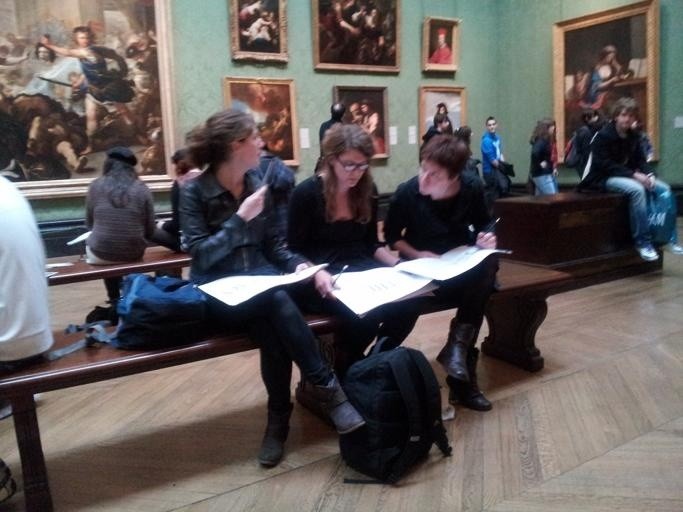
[564,126,589,168]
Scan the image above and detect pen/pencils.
[646,171,654,178]
[321,265,347,299]
[478,217,501,240]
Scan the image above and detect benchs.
[38,241,193,311]
[493,181,668,264]
[0,249,574,510]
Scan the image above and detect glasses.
[336,156,370,172]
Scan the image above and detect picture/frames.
[416,84,470,166]
[421,17,460,75]
[331,84,393,166]
[309,2,401,75]
[550,2,662,170]
[225,0,289,66]
[0,2,184,201]
[224,79,302,168]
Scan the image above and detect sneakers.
[635,244,659,262]
[659,242,683,255]
[0,457,16,506]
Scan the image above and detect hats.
[107,145,138,168]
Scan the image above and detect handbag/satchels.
[44,273,209,360]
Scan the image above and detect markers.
[256,160,276,187]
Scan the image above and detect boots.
[312,374,365,435]
[436,319,480,381]
[446,348,492,412]
[258,399,294,467]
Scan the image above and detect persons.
[150,149,202,252]
[1,176,54,504]
[85,147,154,307]
[292,124,421,377]
[320,0,394,65]
[0,25,149,178]
[384,134,499,411]
[350,100,385,155]
[428,28,451,64]
[530,119,560,195]
[246,144,296,247]
[569,45,630,114]
[456,127,471,171]
[418,115,450,167]
[422,104,454,146]
[481,117,505,199]
[574,110,600,181]
[315,104,346,172]
[238,2,279,53]
[575,97,683,261]
[178,109,364,468]
[630,119,656,166]
